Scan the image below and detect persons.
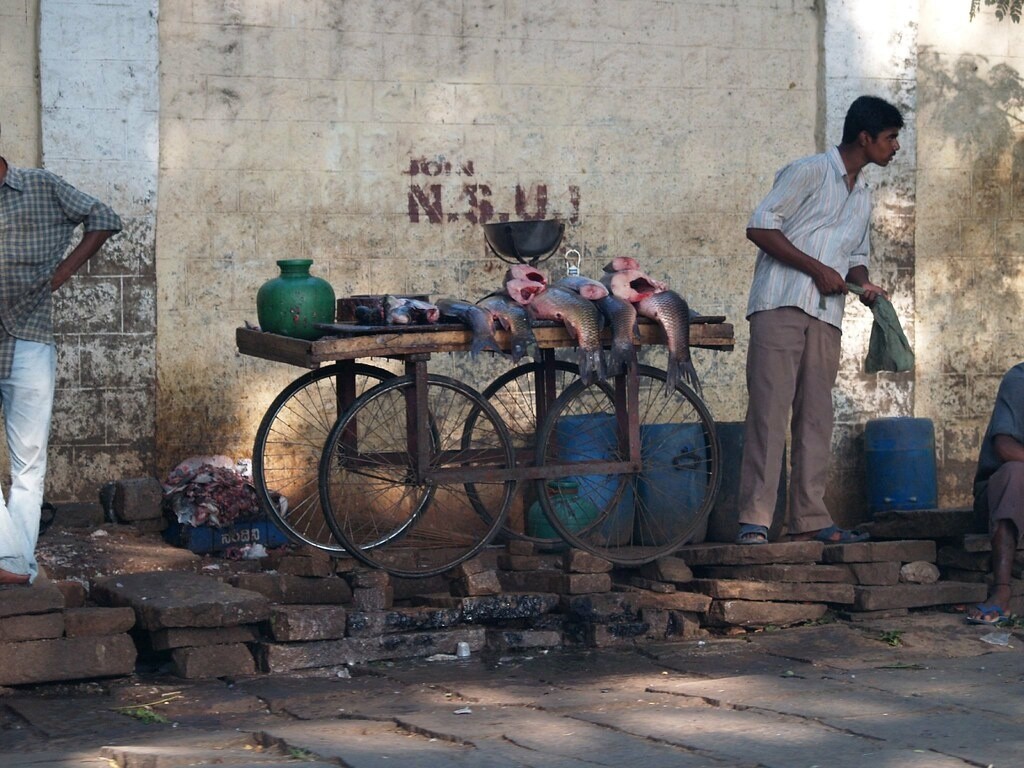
[965,363,1024,624]
[0,155,123,584]
[734,95,905,544]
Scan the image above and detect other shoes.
[0,568,29,583]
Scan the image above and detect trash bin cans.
[635,421,706,550]
[866,416,939,508]
[522,409,636,548]
[713,420,787,539]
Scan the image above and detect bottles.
[256,258,335,341]
[529,481,600,539]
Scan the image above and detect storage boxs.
[161,509,292,554]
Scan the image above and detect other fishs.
[355,258,704,396]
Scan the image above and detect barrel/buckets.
[636,423,709,546]
[864,417,937,514]
[713,420,787,543]
[543,412,634,546]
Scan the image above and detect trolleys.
[235,315,736,578]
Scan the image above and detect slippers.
[966,605,1009,625]
[808,523,871,544]
[736,524,768,544]
